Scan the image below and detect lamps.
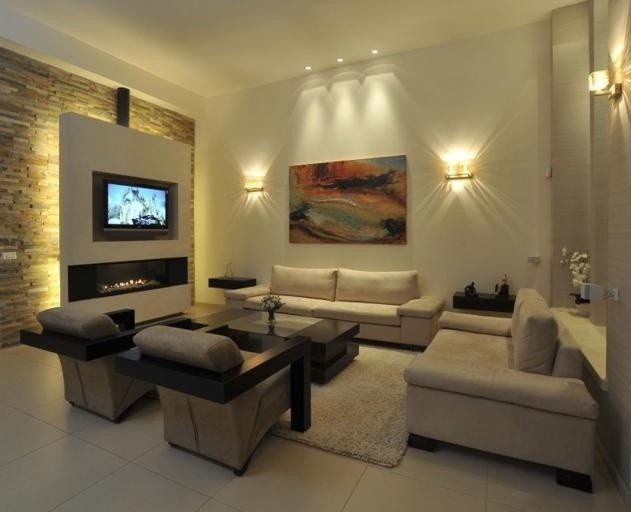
[441,143,478,180]
[241,165,265,193]
[586,67,626,102]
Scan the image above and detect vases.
[260,287,287,321]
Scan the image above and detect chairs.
[20,306,209,425]
[113,322,313,479]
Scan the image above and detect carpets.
[265,339,425,467]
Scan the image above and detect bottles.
[223,263,233,280]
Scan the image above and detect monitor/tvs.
[103,178,169,229]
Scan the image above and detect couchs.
[224,264,448,352]
[403,288,600,494]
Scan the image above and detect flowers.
[558,243,593,301]
